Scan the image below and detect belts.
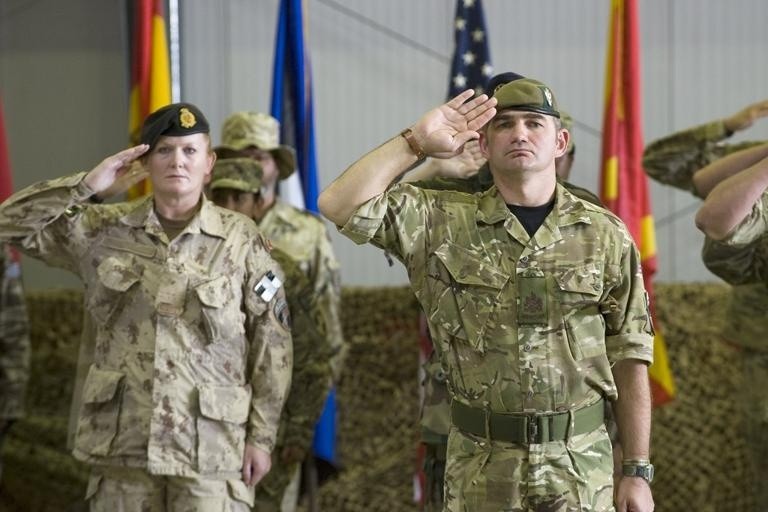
[450,397,607,442]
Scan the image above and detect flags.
[598,0,679,443]
[267,0,343,512]
[122,1,183,200]
[413,1,495,502]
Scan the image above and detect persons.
[400,68,608,511]
[642,101,768,285]
[1,103,343,512]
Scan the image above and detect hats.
[139,103,210,155]
[209,157,263,195]
[489,72,573,152]
[213,112,296,179]
